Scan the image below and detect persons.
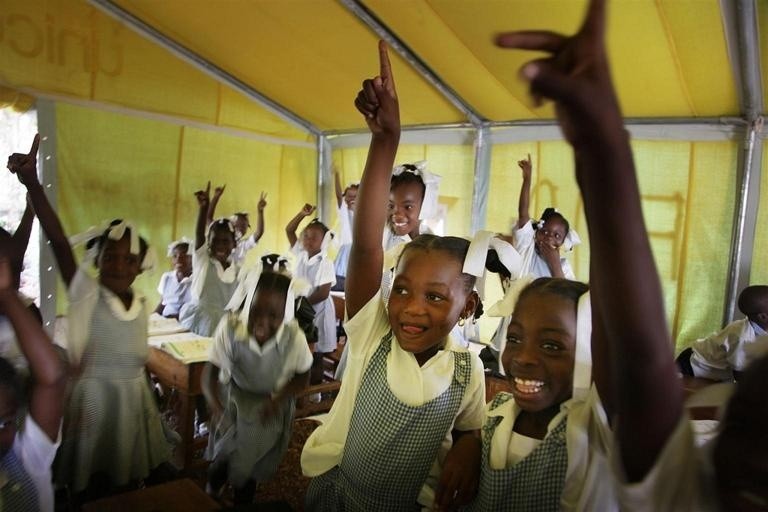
[206,183,268,267]
[676,282,767,381]
[0,193,45,428]
[284,203,339,403]
[6,133,182,498]
[454,277,616,511]
[490,151,581,375]
[381,160,448,309]
[198,252,314,506]
[489,2,768,512]
[300,39,487,512]
[332,170,361,292]
[179,180,248,337]
[154,235,193,320]
[0,248,67,512]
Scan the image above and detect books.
[147,332,211,365]
[147,311,190,336]
